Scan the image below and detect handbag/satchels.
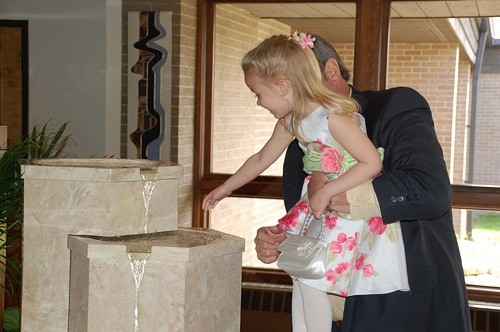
[276,212,329,280]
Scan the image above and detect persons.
[253,31,472,332]
[202,30,410,332]
